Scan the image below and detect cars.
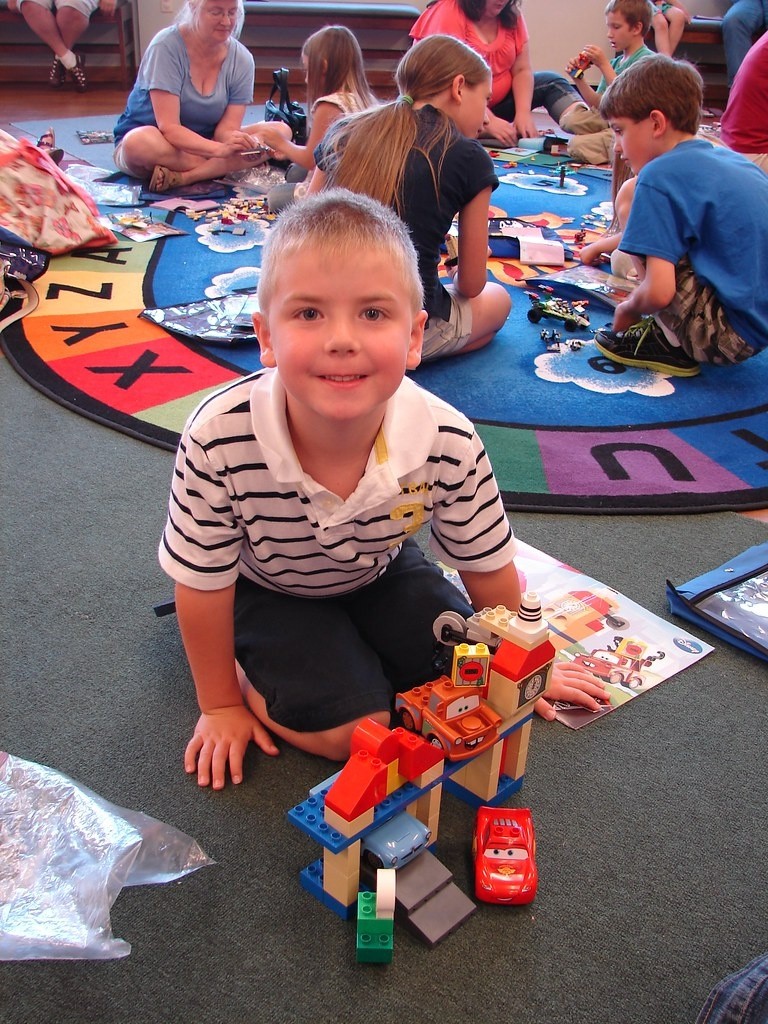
[573,638,652,687]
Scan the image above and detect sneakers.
[594,316,701,376]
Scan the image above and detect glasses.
[197,4,239,20]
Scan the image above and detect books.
[503,227,564,265]
[503,137,546,156]
[150,198,220,213]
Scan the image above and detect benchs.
[0,1,143,89]
[642,16,763,100]
[241,2,421,90]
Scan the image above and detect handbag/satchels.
[0,125,117,335]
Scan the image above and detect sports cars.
[474,805,539,908]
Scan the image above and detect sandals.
[37,127,56,151]
[147,165,180,193]
[69,55,88,93]
[50,57,66,87]
[49,148,64,163]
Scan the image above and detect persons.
[257,27,378,213]
[308,35,512,361]
[649,0,690,57]
[721,31,768,175]
[0,127,118,255]
[565,0,654,164]
[410,0,588,148]
[158,188,611,790]
[595,55,768,377]
[722,0,768,88]
[578,130,730,283]
[7,0,116,92]
[113,0,292,193]
